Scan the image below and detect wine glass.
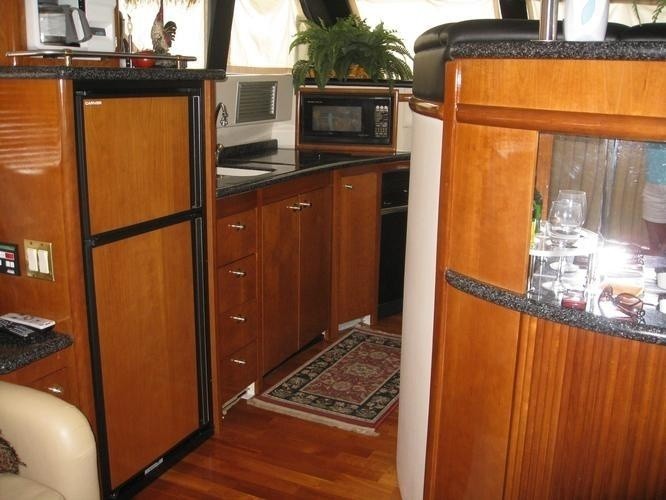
[546,188,588,312]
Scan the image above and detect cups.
[642,184,666,256]
[563,0,611,42]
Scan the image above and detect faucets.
[216,101,229,163]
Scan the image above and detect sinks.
[216,165,276,177]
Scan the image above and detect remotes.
[0,321,37,340]
[0,313,56,335]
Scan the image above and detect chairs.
[0,383,109,500]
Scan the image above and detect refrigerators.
[75,86,214,500]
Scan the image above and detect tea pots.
[38,4,94,48]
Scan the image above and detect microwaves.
[294,81,398,155]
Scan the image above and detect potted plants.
[282,15,412,84]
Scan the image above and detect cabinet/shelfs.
[331,170,378,332]
[216,194,254,418]
[259,183,329,375]
[3,348,75,401]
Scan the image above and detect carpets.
[256,322,400,436]
